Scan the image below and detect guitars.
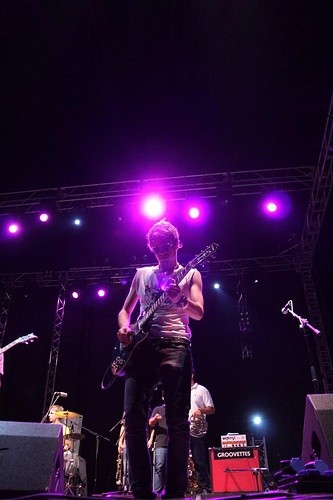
[101,243,220,392]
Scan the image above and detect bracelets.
[176,294,189,310]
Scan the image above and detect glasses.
[153,243,177,252]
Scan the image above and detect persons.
[118,218,204,499]
[149,404,170,500]
[188,368,215,494]
[48,405,71,451]
